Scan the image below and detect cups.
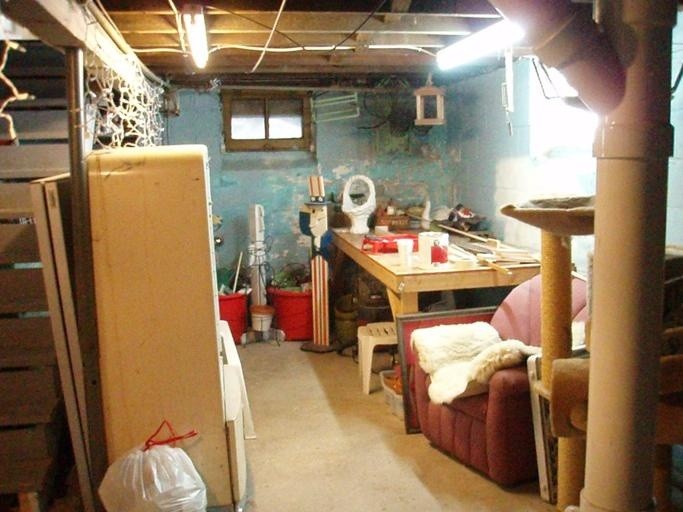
[397,239,414,269]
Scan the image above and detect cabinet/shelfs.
[84,142,247,512]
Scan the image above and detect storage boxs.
[379,368,405,420]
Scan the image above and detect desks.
[331,221,557,385]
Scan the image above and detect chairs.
[393,273,592,495]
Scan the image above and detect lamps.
[435,18,526,72]
[182,3,209,70]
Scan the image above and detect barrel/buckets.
[267,283,313,341]
[218,290,247,342]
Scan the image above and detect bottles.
[430,239,449,263]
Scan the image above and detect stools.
[357,321,399,393]
